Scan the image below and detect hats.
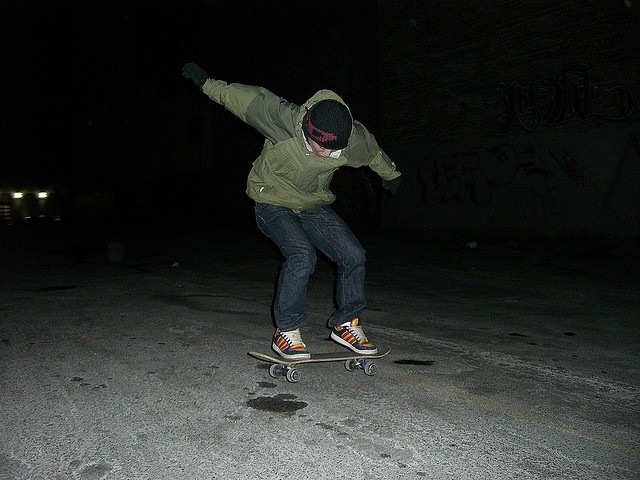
[302,100,352,150]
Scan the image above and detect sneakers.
[273,326,311,361]
[331,318,378,354]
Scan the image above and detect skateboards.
[248,348,390,384]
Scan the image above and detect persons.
[182,62,404,360]
